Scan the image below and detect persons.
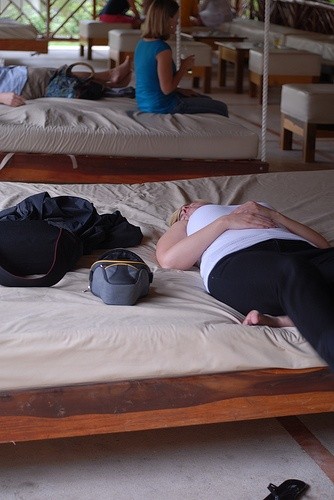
[177,0,231,35]
[96,0,150,31]
[0,55,131,107]
[156,199,334,371]
[134,0,228,118]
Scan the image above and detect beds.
[0,169,334,443]
[0,21,48,55]
[0,96,269,182]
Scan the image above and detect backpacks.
[0,191,145,288]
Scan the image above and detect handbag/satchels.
[43,62,106,100]
[89,246,153,306]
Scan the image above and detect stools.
[167,41,212,95]
[107,30,147,71]
[77,20,133,60]
[214,41,264,94]
[249,48,321,104]
[279,83,334,162]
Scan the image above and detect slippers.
[263,479,310,500]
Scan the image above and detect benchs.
[224,17,334,67]
